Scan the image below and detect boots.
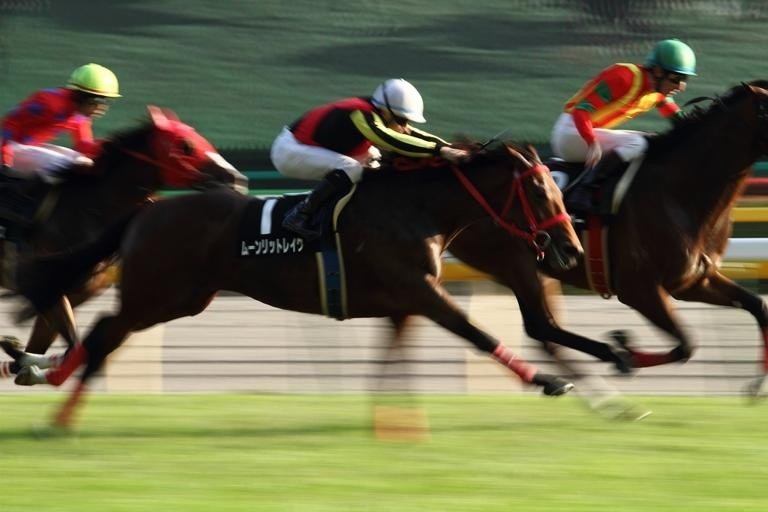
[567,147,630,210]
[281,169,354,242]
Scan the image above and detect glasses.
[394,114,408,127]
[669,76,687,84]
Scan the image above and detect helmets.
[371,78,427,124]
[64,62,124,98]
[645,38,699,76]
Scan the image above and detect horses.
[55,140,581,421]
[1,105,248,387]
[378,79,766,401]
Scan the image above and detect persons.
[267,74,473,254]
[0,59,123,187]
[548,37,701,239]
